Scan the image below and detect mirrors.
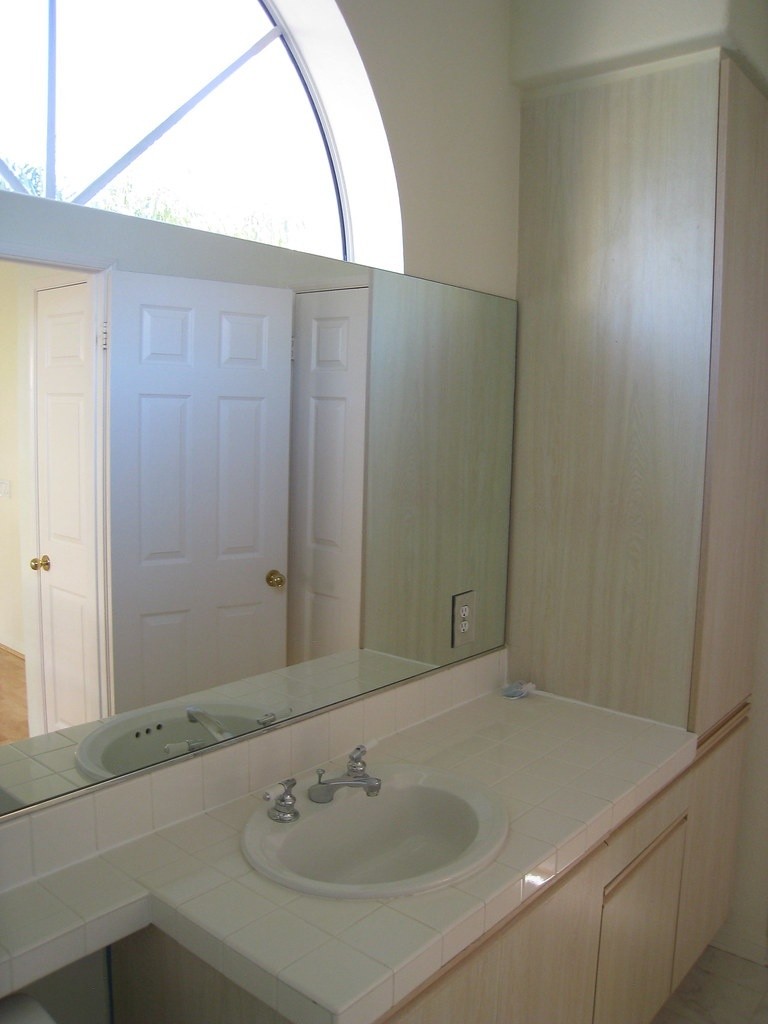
[0,191,521,817]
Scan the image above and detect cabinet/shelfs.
[108,704,753,1024]
[513,45,768,736]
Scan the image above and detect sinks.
[240,781,511,899]
[73,702,274,782]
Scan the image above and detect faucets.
[308,745,381,804]
[185,703,234,743]
